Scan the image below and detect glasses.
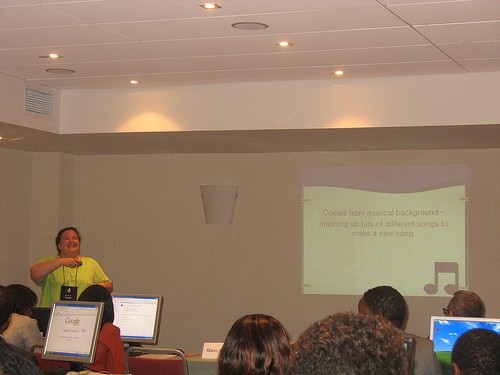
[443,306,448,315]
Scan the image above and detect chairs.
[30,344,189,375]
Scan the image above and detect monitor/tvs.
[111,293,164,356]
[29,307,50,337]
[41,302,105,372]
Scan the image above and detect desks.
[133,353,218,375]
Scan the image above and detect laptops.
[429,316,500,375]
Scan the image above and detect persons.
[0,285,44,375]
[357,286,442,375]
[217,314,296,375]
[77,284,126,375]
[0,284,45,353]
[426,290,485,340]
[285,313,407,375]
[450,328,500,375]
[30,227,113,307]
